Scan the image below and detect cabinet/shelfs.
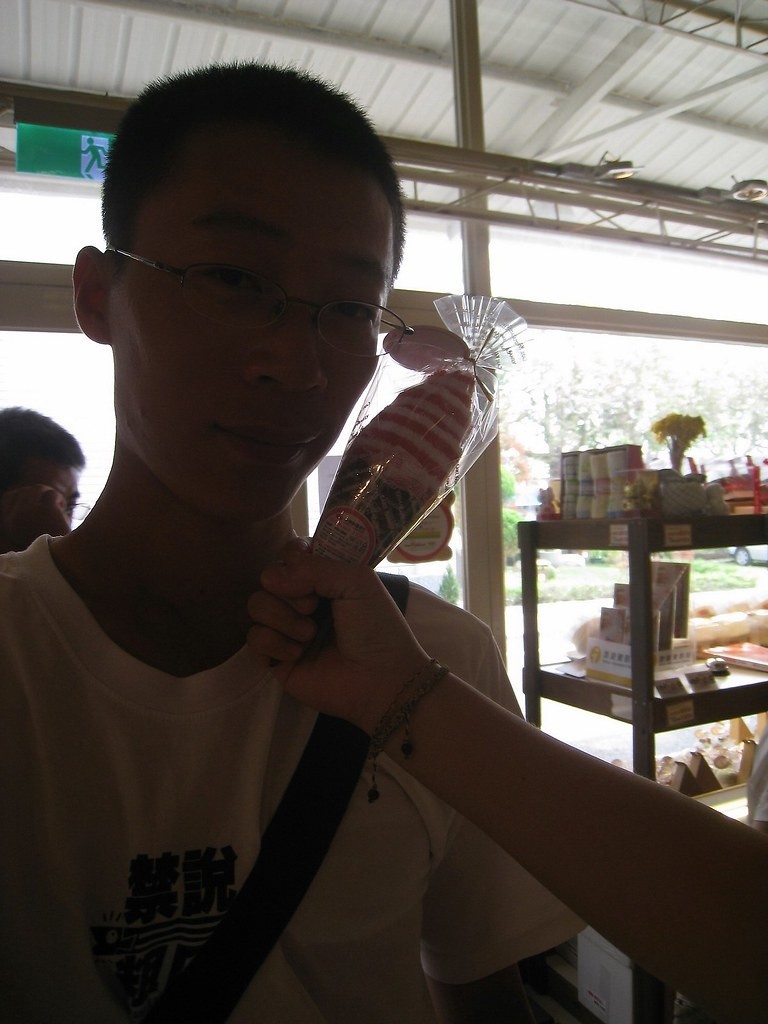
[516,515,768,806]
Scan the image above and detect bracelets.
[365,659,448,802]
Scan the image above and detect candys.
[310,369,475,612]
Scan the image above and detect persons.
[249,537,768,1024]
[0,64,589,1024]
[0,406,91,554]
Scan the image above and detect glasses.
[105,244,415,358]
[9,498,91,521]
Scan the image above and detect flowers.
[652,412,706,470]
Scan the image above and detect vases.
[659,469,707,514]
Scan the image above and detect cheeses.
[656,711,768,797]
